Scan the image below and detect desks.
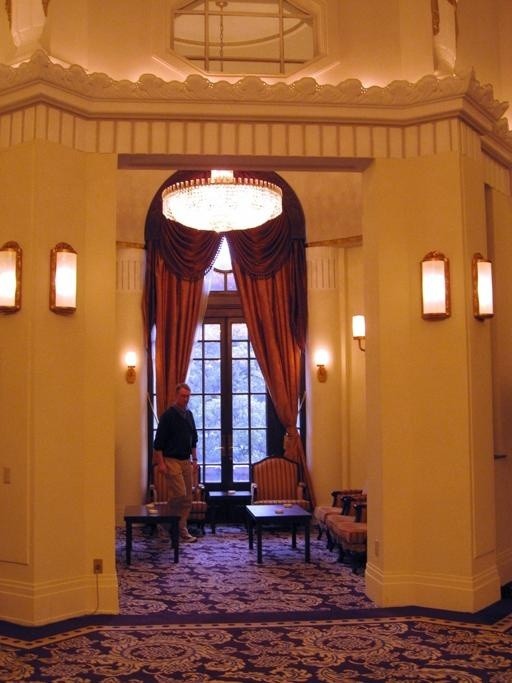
[120,503,183,565]
[204,488,253,535]
[243,500,316,565]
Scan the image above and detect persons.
[154,383,200,543]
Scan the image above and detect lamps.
[44,239,83,316]
[0,238,24,317]
[311,345,332,383]
[468,248,500,322]
[120,348,139,383]
[157,165,288,235]
[350,312,370,354]
[417,247,453,324]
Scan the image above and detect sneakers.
[179,533,197,542]
[158,524,170,543]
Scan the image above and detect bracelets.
[192,458,197,461]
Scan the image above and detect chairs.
[324,498,370,571]
[149,457,208,539]
[311,485,365,549]
[240,453,312,519]
